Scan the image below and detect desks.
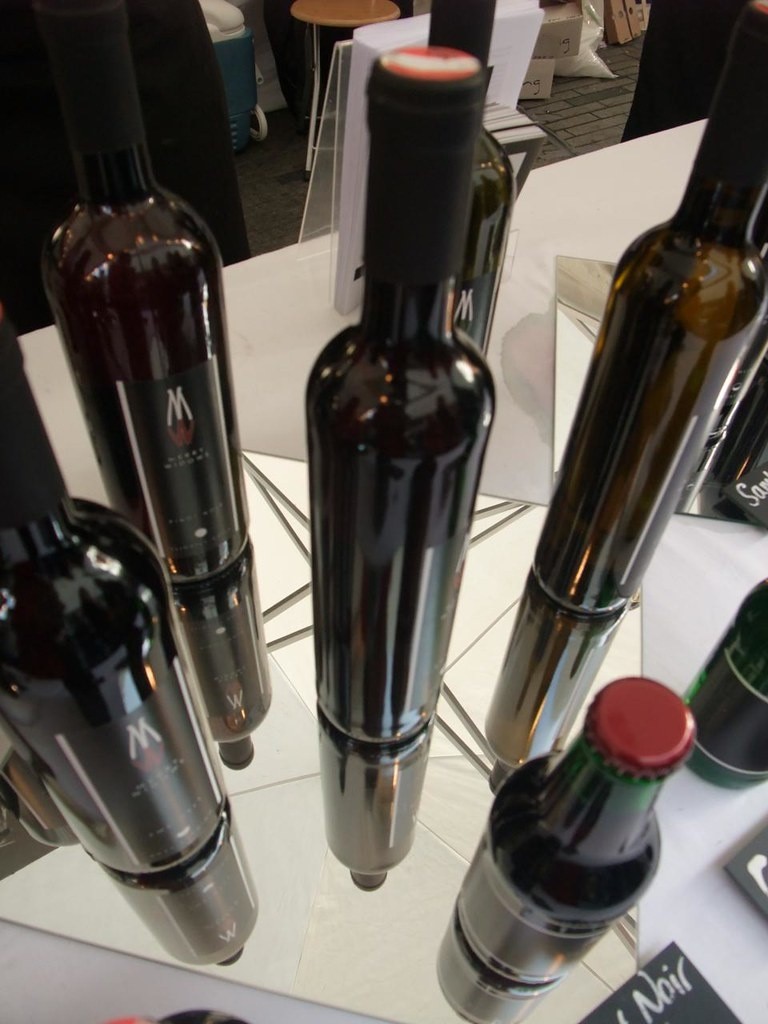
[0,115,765,1023]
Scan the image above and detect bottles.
[486,563,633,799]
[455,677,696,986]
[104,1009,247,1024]
[428,0,516,365]
[716,347,768,485]
[304,46,495,746]
[0,294,225,879]
[102,797,259,966]
[435,902,572,1024]
[165,538,273,770]
[36,0,249,587]
[533,0,768,617]
[315,704,436,892]
[682,576,768,792]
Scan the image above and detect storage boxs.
[200,0,259,153]
[518,1,642,99]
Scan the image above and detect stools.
[290,0,400,182]
